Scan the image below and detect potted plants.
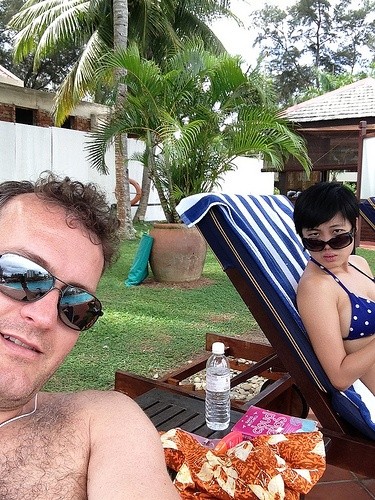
[83,35,313,281]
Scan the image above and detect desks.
[131,387,331,500]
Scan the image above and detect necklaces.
[0,393,41,432]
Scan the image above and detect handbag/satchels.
[125,233,154,285]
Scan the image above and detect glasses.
[300,225,355,252]
[0,253,103,331]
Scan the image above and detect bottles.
[205,343,230,431]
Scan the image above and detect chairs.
[116,194,375,443]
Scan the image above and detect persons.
[293,182,375,397]
[0,171,183,500]
